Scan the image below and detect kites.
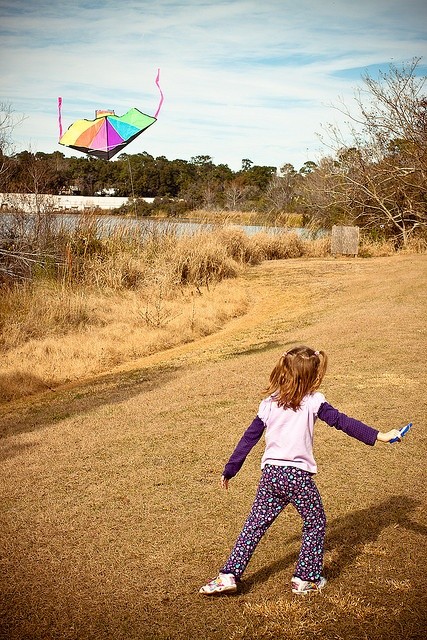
[59,108,157,163]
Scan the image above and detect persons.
[198,345,404,599]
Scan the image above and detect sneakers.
[291,576,327,594]
[199,572,238,594]
